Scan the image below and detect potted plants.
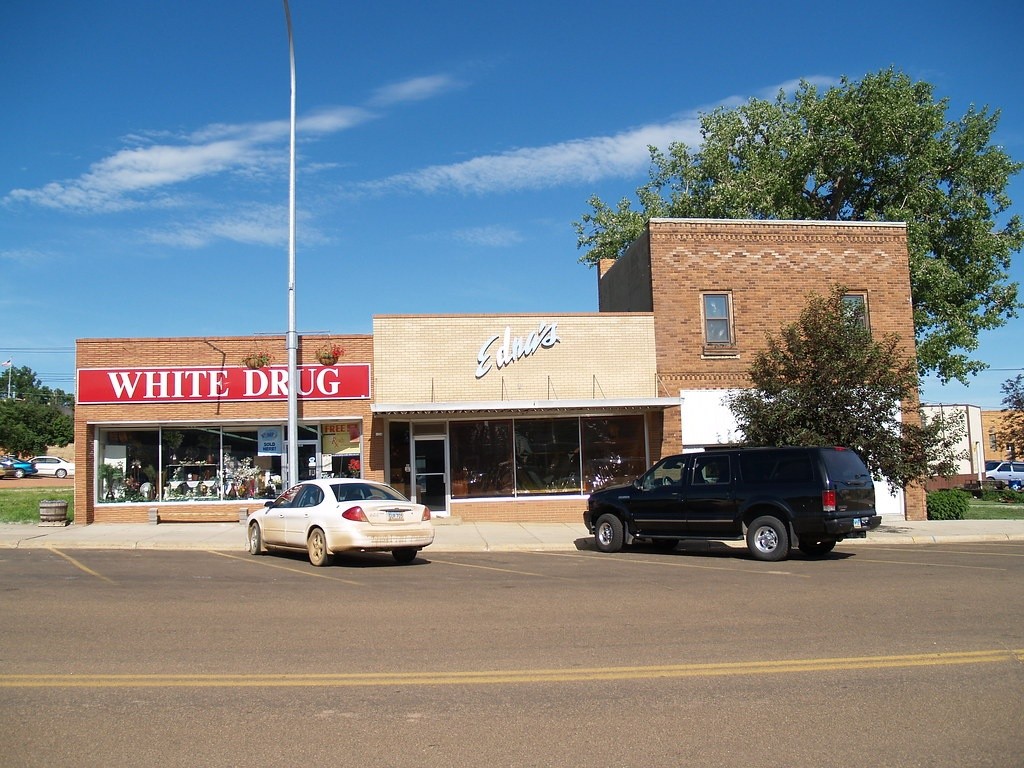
[199,433,218,463]
[258,485,275,496]
[168,468,215,480]
[163,432,184,464]
[143,465,156,500]
[99,463,123,501]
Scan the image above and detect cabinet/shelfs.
[165,464,219,500]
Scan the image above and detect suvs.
[985,462,1024,488]
[553,441,646,485]
[582,446,883,562]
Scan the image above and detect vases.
[228,482,240,500]
[351,473,357,478]
[245,359,264,369]
[319,356,339,366]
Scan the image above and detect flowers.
[222,453,261,482]
[235,349,275,367]
[347,458,360,472]
[314,343,346,359]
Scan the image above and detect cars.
[1,456,39,478]
[0,457,16,480]
[245,477,435,567]
[400,473,445,503]
[26,456,75,478]
